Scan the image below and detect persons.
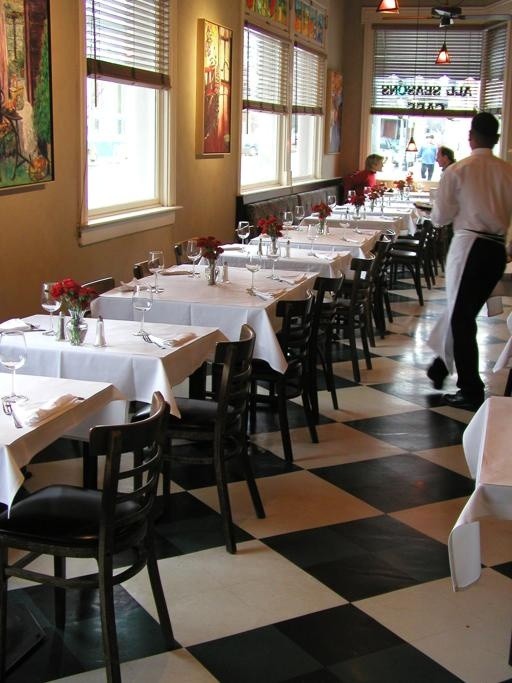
[416,111,511,412]
[416,133,437,181]
[435,145,457,171]
[344,153,384,204]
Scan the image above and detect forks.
[247,288,268,302]
[2,402,24,429]
[143,333,165,350]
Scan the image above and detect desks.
[448,392,512,590]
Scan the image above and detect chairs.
[130,324,267,555]
[131,256,167,280]
[173,239,202,266]
[1,392,174,683]
[236,179,456,467]
[81,277,117,319]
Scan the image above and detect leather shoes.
[444,387,488,411]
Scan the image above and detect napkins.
[11,394,82,427]
[150,331,197,348]
[120,280,145,294]
[160,269,194,275]
[0,317,39,334]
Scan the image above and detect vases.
[203,260,219,285]
[65,308,89,347]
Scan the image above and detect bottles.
[93,316,108,348]
[54,309,67,342]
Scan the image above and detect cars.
[242,132,259,155]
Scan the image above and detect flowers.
[194,236,225,261]
[51,277,87,301]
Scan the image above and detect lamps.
[429,1,460,66]
[406,119,420,153]
[375,0,400,13]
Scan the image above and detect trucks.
[380,115,416,171]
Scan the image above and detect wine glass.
[40,281,65,337]
[0,329,27,404]
[129,282,154,337]
[145,181,424,296]
